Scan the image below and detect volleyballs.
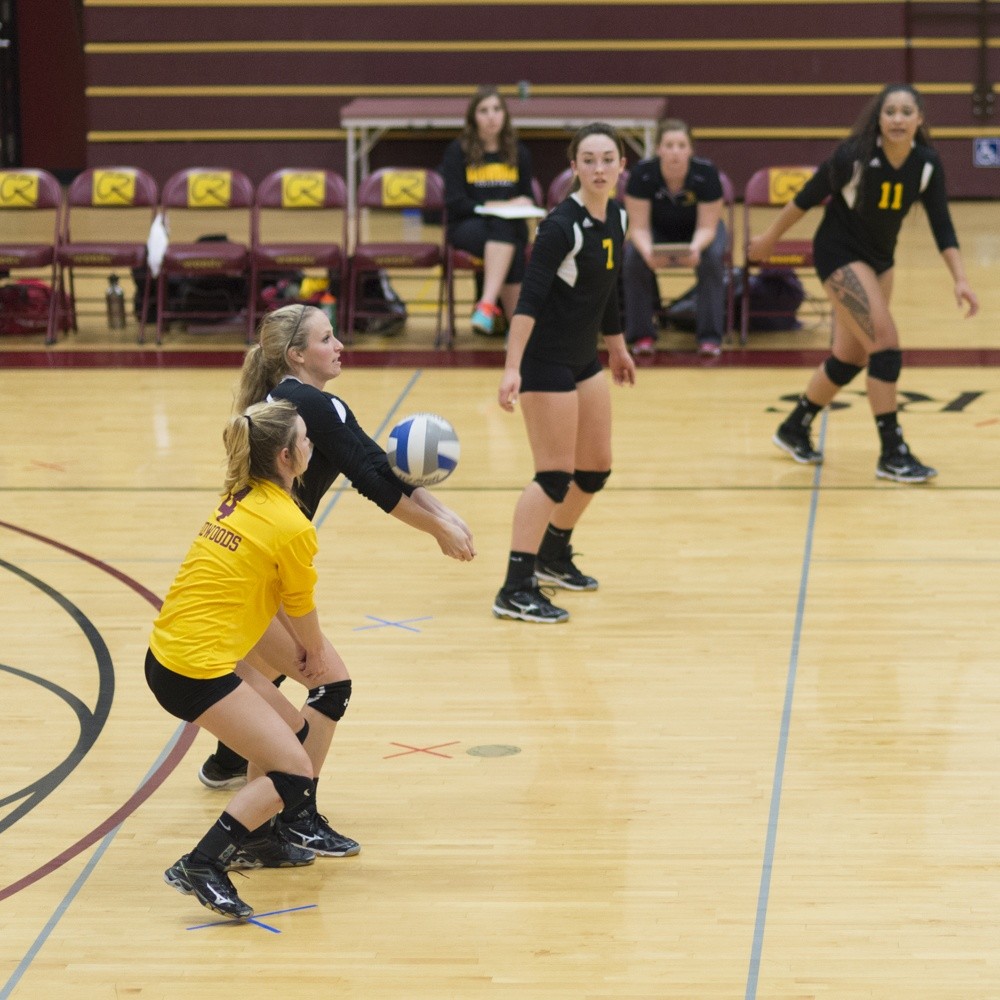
[386,411,462,488]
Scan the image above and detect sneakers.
[224,829,317,870]
[534,544,599,592]
[199,754,247,792]
[471,301,501,334]
[273,812,360,857]
[875,444,938,484]
[164,854,255,920]
[493,575,569,624]
[772,422,822,465]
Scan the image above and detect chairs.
[50,163,157,347]
[664,167,741,345]
[443,180,543,353]
[743,162,843,355]
[247,166,350,346]
[349,166,451,351]
[549,166,632,209]
[154,165,256,344]
[0,165,64,348]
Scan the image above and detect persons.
[438,84,539,336]
[143,396,331,922]
[624,118,727,356]
[196,302,478,859]
[491,120,637,625]
[746,81,979,483]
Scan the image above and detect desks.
[329,81,676,196]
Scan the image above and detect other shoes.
[633,337,655,355]
[697,343,719,355]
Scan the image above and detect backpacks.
[325,256,409,337]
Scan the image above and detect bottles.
[105,273,127,331]
[319,292,337,340]
